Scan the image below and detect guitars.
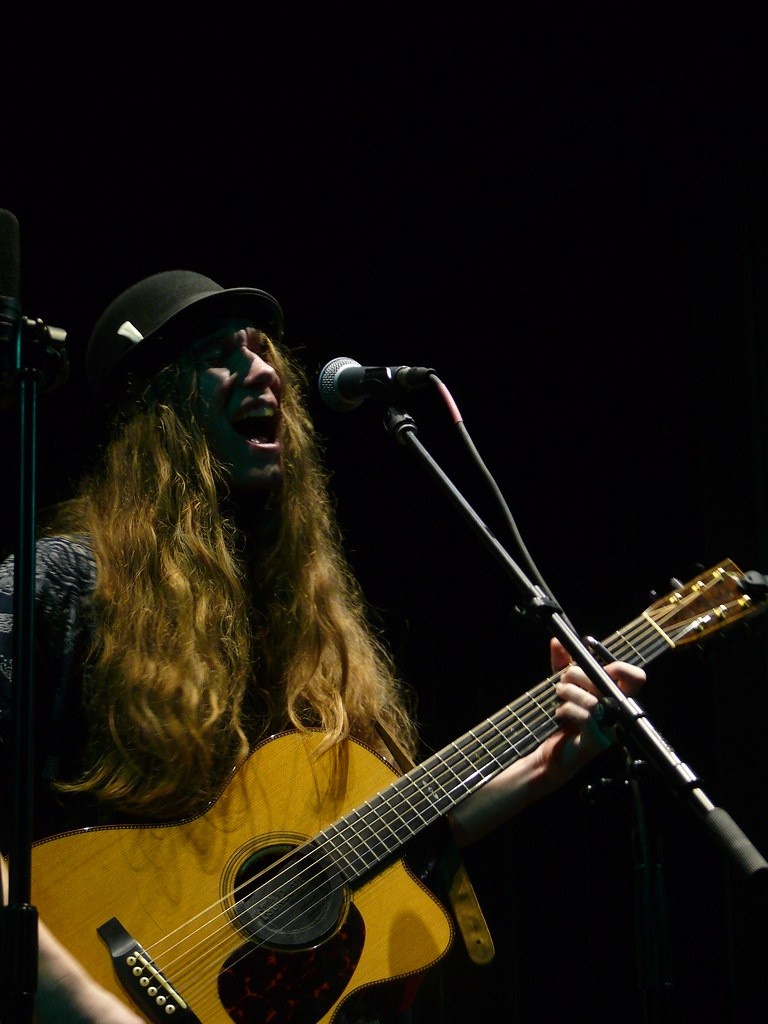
[2,559,768,1024]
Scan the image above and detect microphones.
[319,357,435,412]
[0,208,22,341]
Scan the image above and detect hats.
[80,266,288,411]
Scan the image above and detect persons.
[2,273,645,1024]
